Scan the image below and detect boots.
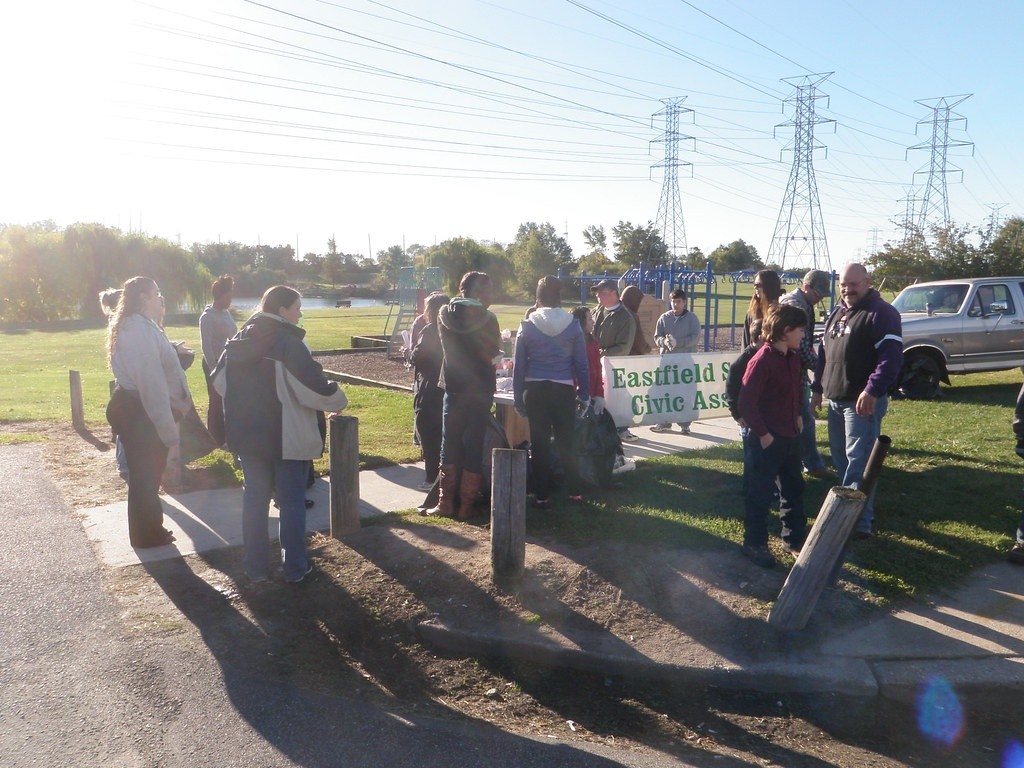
[425,463,457,516]
[458,468,481,520]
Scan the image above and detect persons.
[512,274,650,508]
[649,289,700,434]
[100,277,191,548]
[724,270,833,567]
[209,285,348,583]
[401,271,501,519]
[809,264,902,539]
[200,279,236,448]
[1008,381,1024,565]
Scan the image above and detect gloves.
[1015,444,1024,459]
[513,407,528,418]
[576,394,590,418]
[593,395,605,416]
[664,333,677,351]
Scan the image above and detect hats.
[591,279,619,293]
[804,269,833,297]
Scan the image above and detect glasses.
[144,292,161,299]
[839,278,867,290]
[754,284,764,289]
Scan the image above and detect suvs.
[890,277,1024,399]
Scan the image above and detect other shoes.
[289,564,313,582]
[807,469,837,480]
[570,491,583,501]
[303,500,314,510]
[418,480,434,492]
[781,540,802,556]
[133,529,176,546]
[533,495,549,509]
[1009,542,1024,563]
[852,531,869,542]
[245,571,267,583]
[743,538,775,567]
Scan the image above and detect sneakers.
[617,430,638,442]
[649,424,672,433]
[681,425,690,435]
